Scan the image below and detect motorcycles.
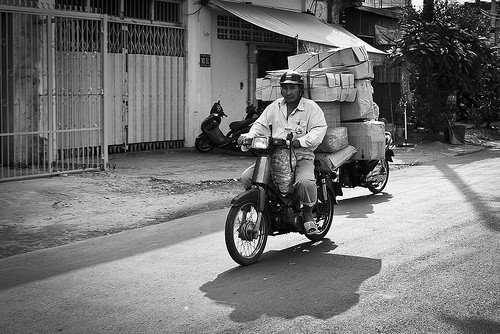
[225,135,342,266]
[194,99,273,157]
[245,103,263,120]
[333,132,395,196]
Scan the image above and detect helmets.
[279,72,304,84]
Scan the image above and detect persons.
[237,70,329,235]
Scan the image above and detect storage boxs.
[255,44,386,172]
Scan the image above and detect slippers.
[303,221,317,235]
